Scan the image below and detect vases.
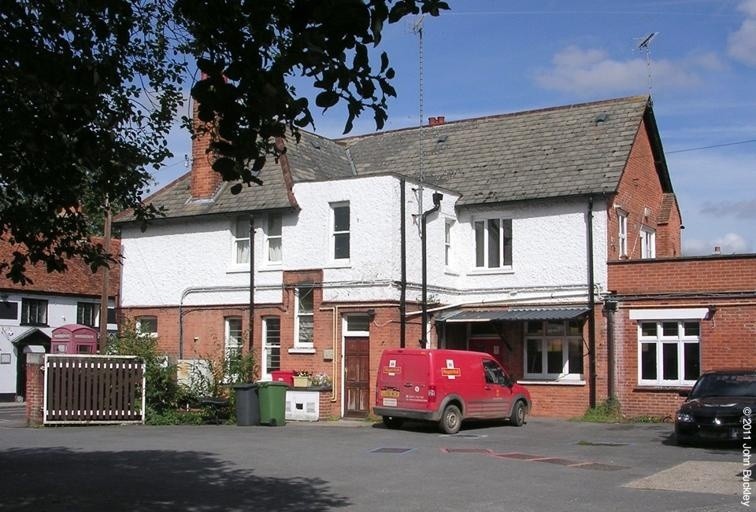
[291,376,312,388]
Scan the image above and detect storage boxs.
[270,371,293,387]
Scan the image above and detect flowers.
[294,368,312,378]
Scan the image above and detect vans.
[373,348,531,433]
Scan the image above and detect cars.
[673,366,756,450]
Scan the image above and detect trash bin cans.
[257,382,288,426]
[233,384,260,426]
